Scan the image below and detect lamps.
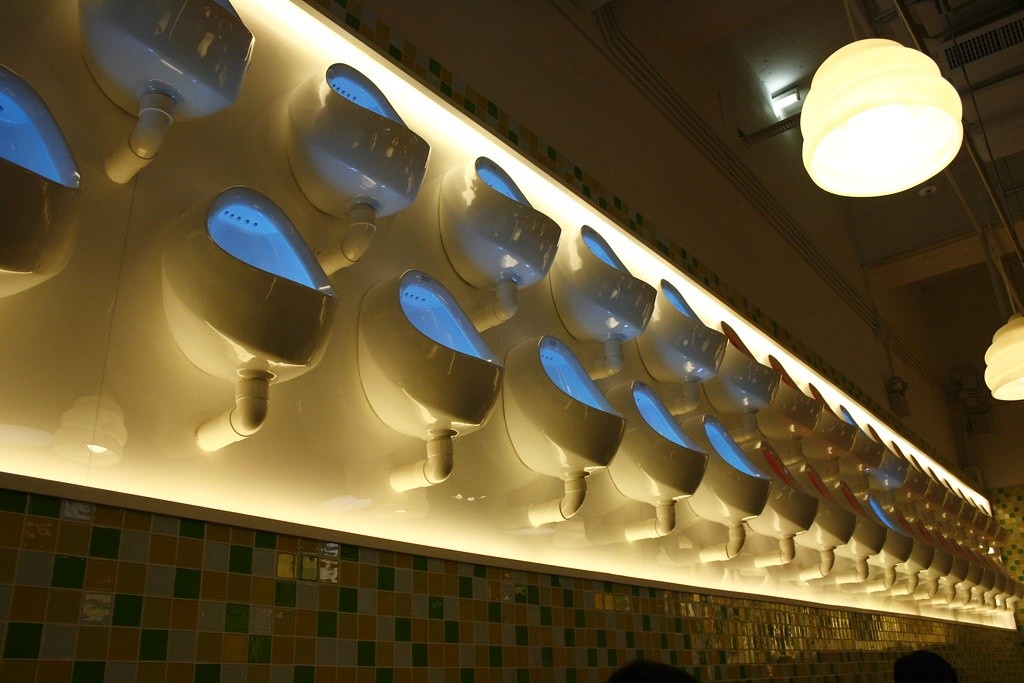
[742,37,966,199]
[982,207,1024,402]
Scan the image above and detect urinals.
[1,0,1022,613]
